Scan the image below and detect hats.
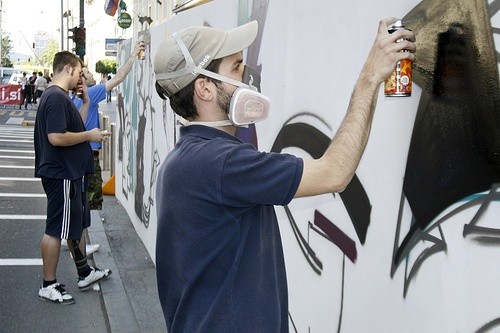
[154,20,258,97]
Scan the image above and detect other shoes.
[70,244,100,260]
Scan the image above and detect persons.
[33,51,112,305]
[153,16,417,333]
[43,71,52,83]
[34,71,47,99]
[29,72,37,104]
[18,72,28,110]
[107,75,111,103]
[70,39,145,260]
[100,76,107,83]
[50,73,53,80]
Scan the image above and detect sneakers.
[77,267,112,291]
[38,282,75,304]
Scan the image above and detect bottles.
[138,41,145,60]
[383,21,412,98]
